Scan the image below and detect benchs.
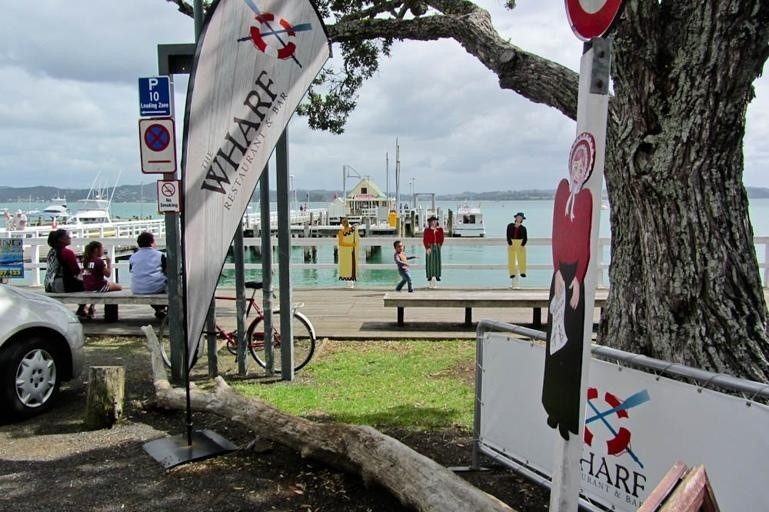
[383,291,609,325]
[43,293,168,324]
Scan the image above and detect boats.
[457,199,484,224]
[2,197,111,226]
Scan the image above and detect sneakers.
[77,314,96,320]
[155,310,167,317]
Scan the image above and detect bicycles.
[157,257,314,375]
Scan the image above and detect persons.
[81,239,123,292]
[128,231,168,319]
[506,212,528,278]
[42,228,97,321]
[539,132,597,441]
[337,216,361,281]
[423,216,444,282]
[392,240,422,292]
[15,209,27,238]
[4,211,16,237]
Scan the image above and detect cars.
[0,283,82,417]
